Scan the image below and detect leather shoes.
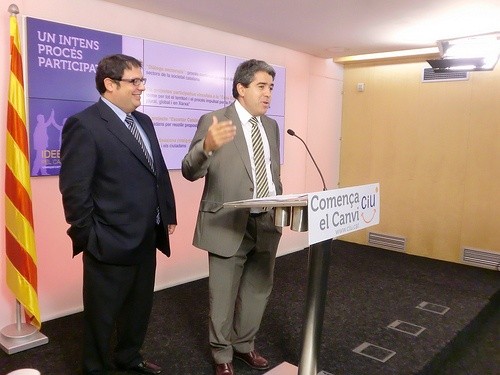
[233,351,270,370]
[134,360,161,375]
[215,363,235,375]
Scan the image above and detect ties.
[248,117,270,211]
[125,114,160,225]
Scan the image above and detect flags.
[5,11,42,329]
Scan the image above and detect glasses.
[113,78,147,86]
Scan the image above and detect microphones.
[287,129,327,191]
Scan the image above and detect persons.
[60,54,178,375]
[181,59,283,375]
